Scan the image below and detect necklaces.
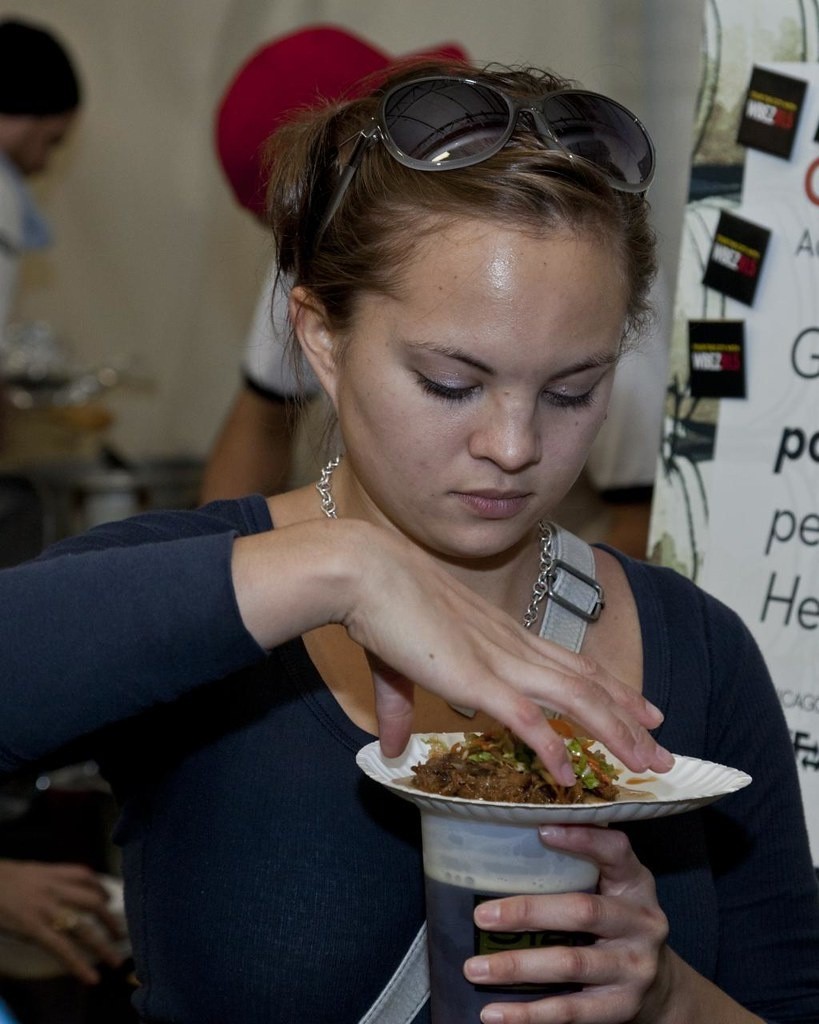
[316,454,553,718]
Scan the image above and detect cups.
[423,812,602,1024]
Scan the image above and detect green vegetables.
[464,722,620,791]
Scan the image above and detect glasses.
[299,75,655,290]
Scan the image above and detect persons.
[0,16,81,256]
[198,25,470,516]
[0,860,126,985]
[0,59,819,1024]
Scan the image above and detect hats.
[215,23,471,228]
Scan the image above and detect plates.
[356,731,756,822]
